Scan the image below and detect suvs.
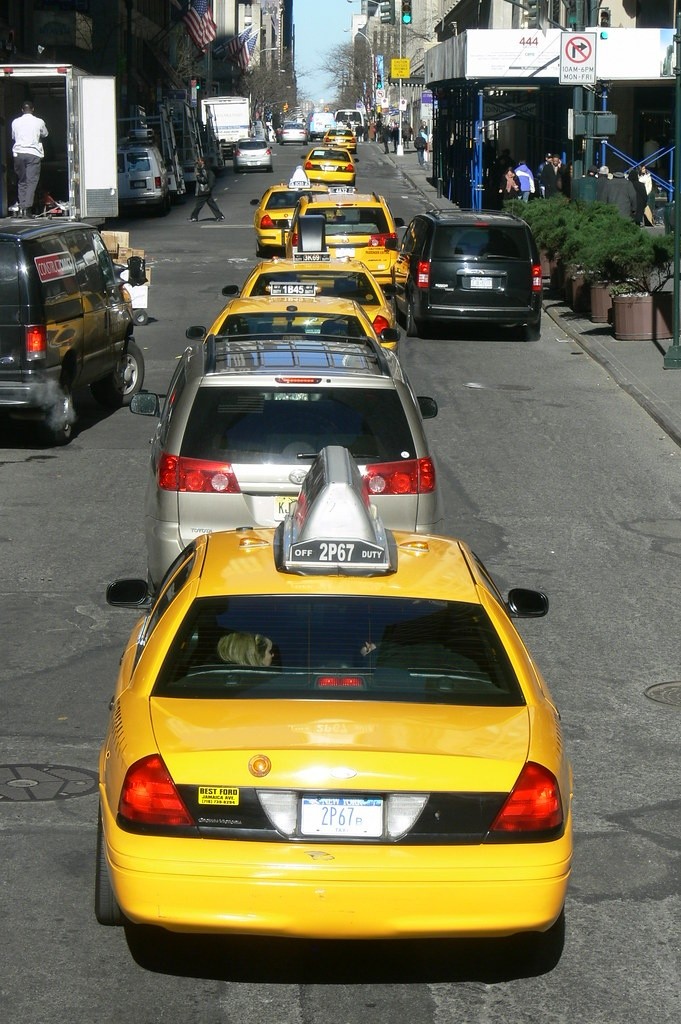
[395,208,544,340]
[128,332,440,597]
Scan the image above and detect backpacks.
[206,168,216,188]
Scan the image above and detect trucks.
[200,96,250,159]
[0,64,119,229]
[132,88,227,208]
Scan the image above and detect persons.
[497,152,655,230]
[419,129,428,162]
[187,158,225,222]
[11,101,49,219]
[414,133,427,165]
[355,118,412,154]
[211,632,275,687]
[419,121,428,151]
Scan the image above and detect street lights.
[343,28,379,143]
[347,0,404,156]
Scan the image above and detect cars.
[186,281,398,356]
[251,164,330,257]
[94,445,573,970]
[279,122,307,146]
[219,253,398,353]
[276,186,400,297]
[322,125,358,154]
[232,138,274,174]
[301,142,359,187]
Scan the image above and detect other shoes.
[215,216,225,222]
[188,218,198,221]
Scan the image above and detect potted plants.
[500,191,681,342]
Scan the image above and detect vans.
[302,112,337,142]
[116,129,173,218]
[0,217,148,447]
[334,109,365,137]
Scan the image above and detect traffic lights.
[402,0,412,24]
[601,10,611,27]
[283,105,288,113]
[377,74,381,88]
[380,0,396,25]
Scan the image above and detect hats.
[545,153,552,158]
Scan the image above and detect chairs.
[237,319,273,341]
[320,320,349,343]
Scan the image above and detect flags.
[230,26,258,70]
[182,0,217,54]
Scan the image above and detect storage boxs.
[100,230,152,310]
